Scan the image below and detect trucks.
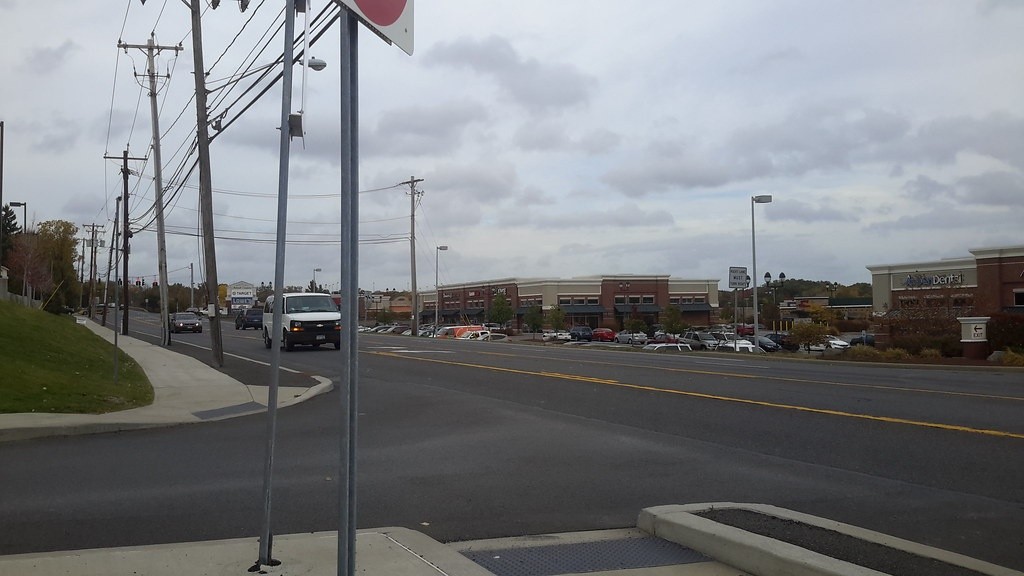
[436,327,484,338]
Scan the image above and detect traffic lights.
[142,279,145,286]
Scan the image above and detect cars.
[169,312,203,334]
[235,308,263,331]
[764,332,800,352]
[570,327,592,342]
[79,307,104,315]
[715,344,766,353]
[677,331,719,351]
[742,336,783,352]
[62,307,75,315]
[642,343,692,351]
[477,333,509,343]
[708,332,752,345]
[614,330,647,345]
[357,324,452,337]
[481,323,506,331]
[804,335,850,351]
[850,334,875,347]
[454,331,490,340]
[654,330,680,340]
[687,324,765,337]
[647,334,678,344]
[542,330,571,342]
[592,328,616,342]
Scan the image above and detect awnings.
[442,310,458,315]
[419,310,435,315]
[457,309,484,314]
[560,305,608,313]
[615,305,661,312]
[514,307,542,314]
[670,303,713,311]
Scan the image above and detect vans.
[262,293,341,352]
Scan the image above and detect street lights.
[619,281,630,318]
[10,202,26,234]
[436,245,448,324]
[196,58,326,364]
[386,288,395,311]
[763,271,786,352]
[742,275,751,339]
[313,268,321,293]
[826,281,837,321]
[750,195,772,352]
